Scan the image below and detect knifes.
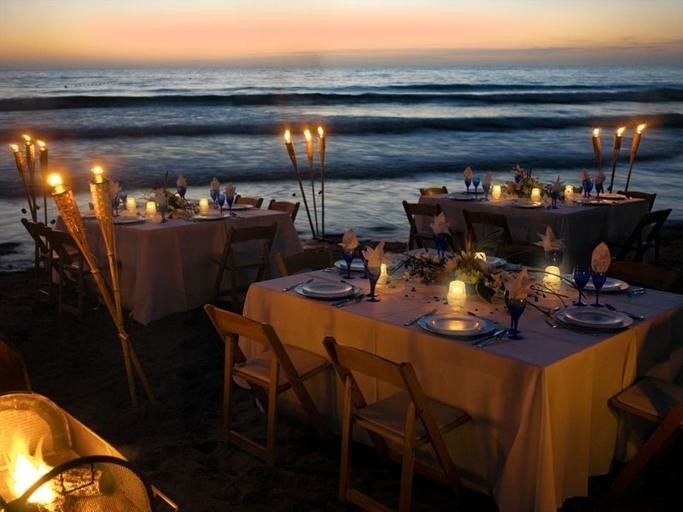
[405,308,437,326]
[281,278,314,292]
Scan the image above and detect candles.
[472,251,487,262]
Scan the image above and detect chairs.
[515,242,564,268]
[607,375,683,503]
[321,335,472,512]
[274,244,334,277]
[203,303,333,470]
[401,184,676,265]
[587,261,678,290]
[20,194,301,324]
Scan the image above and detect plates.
[294,282,361,301]
[553,307,633,331]
[560,277,630,293]
[73,175,634,276]
[418,314,497,336]
